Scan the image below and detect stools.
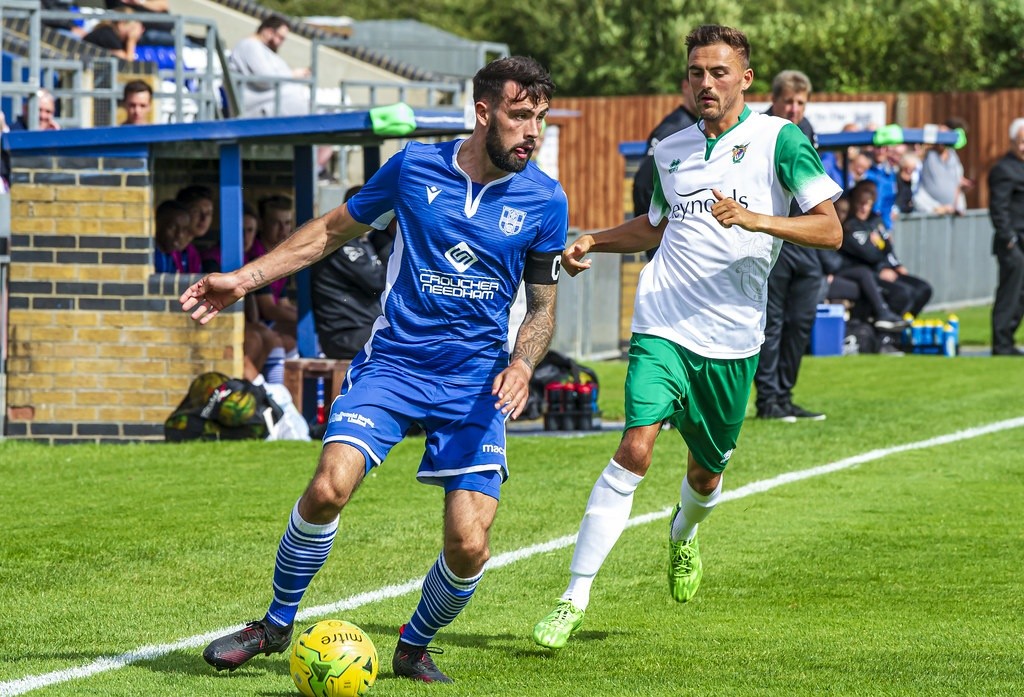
[283,358,352,414]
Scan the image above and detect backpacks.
[161,372,285,441]
[514,348,599,419]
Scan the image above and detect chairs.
[132,45,231,120]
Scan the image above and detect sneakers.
[392,624,452,684]
[667,502,704,603]
[202,615,293,671]
[534,598,585,649]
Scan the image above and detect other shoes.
[789,404,826,421]
[756,408,797,423]
[993,346,1024,356]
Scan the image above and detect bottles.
[905,313,960,358]
[544,380,599,428]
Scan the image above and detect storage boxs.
[811,303,845,355]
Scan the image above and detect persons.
[817,119,972,353]
[156,56,566,680]
[530,24,842,653]
[119,80,154,125]
[987,117,1024,356]
[43,0,225,63]
[227,16,351,120]
[0,89,61,188]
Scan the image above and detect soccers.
[165,373,265,439]
[289,619,379,697]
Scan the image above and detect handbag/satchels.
[250,375,314,441]
